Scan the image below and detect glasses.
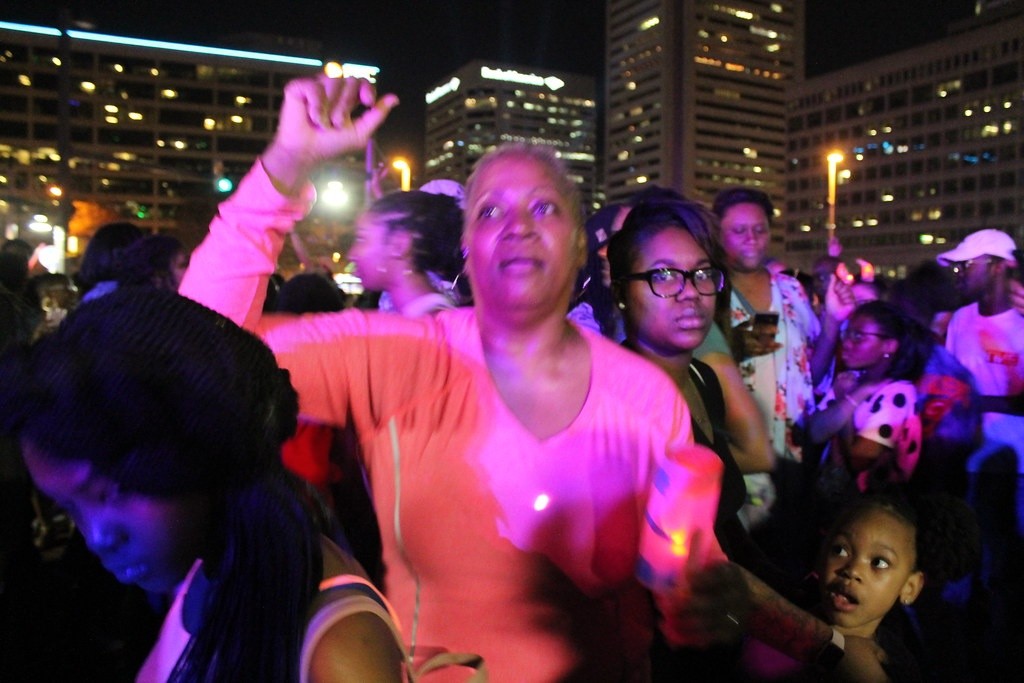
[951,258,998,272]
[844,328,890,344]
[624,264,725,298]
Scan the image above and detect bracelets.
[846,395,858,407]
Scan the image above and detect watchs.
[808,628,845,674]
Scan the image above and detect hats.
[937,229,1016,268]
[419,179,467,210]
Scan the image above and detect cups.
[637,438,722,594]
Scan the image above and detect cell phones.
[752,312,780,341]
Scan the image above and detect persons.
[178,76,742,683]
[0,222,191,357]
[739,478,953,683]
[606,190,888,683]
[264,179,1024,601]
[0,286,412,683]
